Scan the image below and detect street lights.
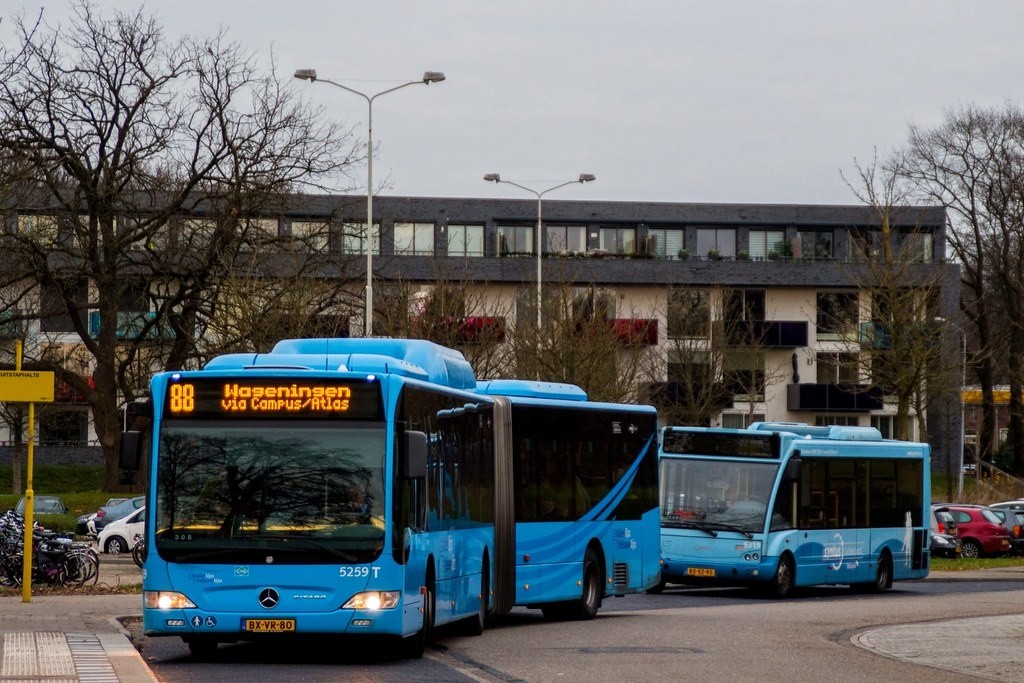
[295,68,447,340]
[932,317,966,500]
[483,172,598,382]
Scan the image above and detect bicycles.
[0,509,100,591]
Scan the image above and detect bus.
[118,339,663,665]
[645,420,932,600]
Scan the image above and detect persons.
[341,439,591,526]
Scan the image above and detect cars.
[930,497,1024,560]
[9,495,69,516]
[75,495,146,556]
